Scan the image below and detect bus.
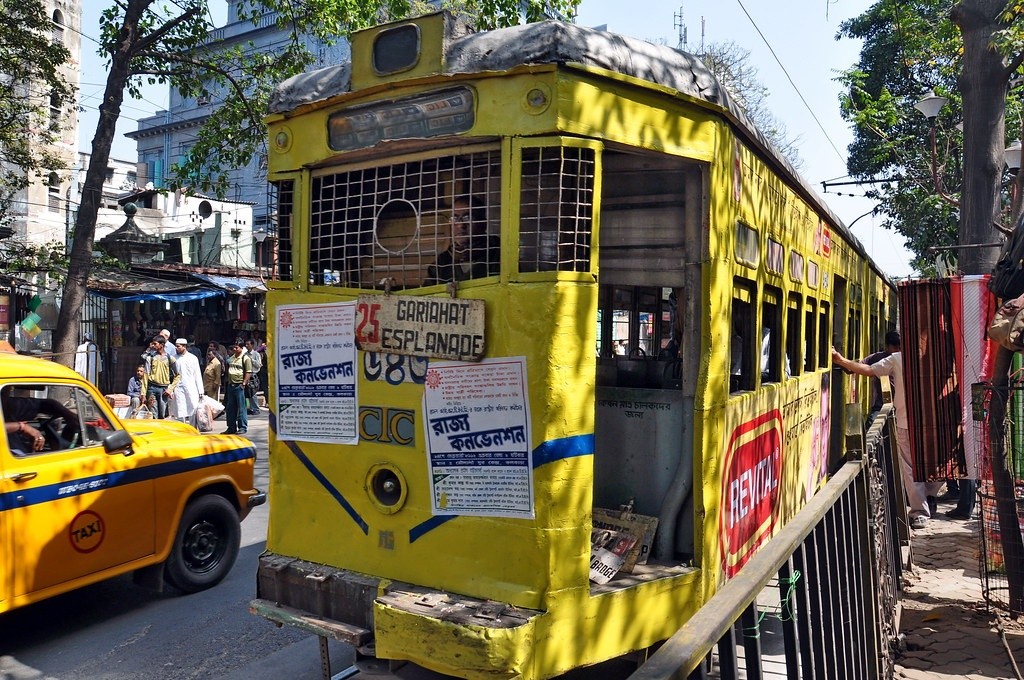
[246,6,901,680]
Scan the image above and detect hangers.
[899,253,965,282]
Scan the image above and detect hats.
[84,332,92,341]
[176,338,187,345]
[161,329,171,338]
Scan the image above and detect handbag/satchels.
[195,394,225,432]
[246,378,260,398]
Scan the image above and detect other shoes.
[909,515,928,529]
[945,508,971,520]
[234,429,247,434]
[926,495,937,515]
[247,410,260,415]
[220,428,237,435]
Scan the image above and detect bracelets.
[18,422,26,434]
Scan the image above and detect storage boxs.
[105,394,152,420]
[257,391,266,406]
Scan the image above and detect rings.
[35,437,43,440]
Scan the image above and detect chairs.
[595,349,682,390]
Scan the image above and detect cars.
[0,349,266,620]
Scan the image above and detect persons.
[832,331,976,528]
[1,385,78,457]
[732,303,793,381]
[809,373,831,497]
[423,195,501,287]
[127,329,268,435]
[75,333,103,387]
[613,286,684,372]
